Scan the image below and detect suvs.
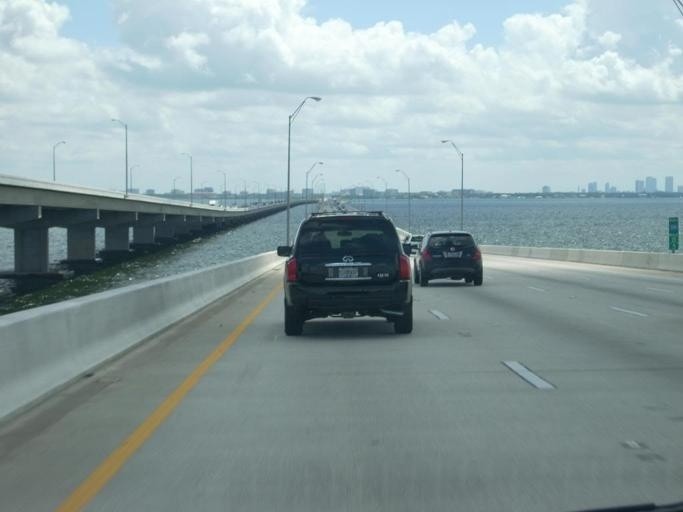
[274,208,416,336]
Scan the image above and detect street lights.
[350,172,391,216]
[50,136,73,186]
[281,93,326,205]
[439,138,466,234]
[302,158,328,220]
[110,114,132,196]
[169,151,295,211]
[393,167,414,236]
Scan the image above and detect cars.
[401,232,425,258]
[330,192,351,215]
[408,229,486,290]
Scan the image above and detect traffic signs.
[668,217,679,251]
